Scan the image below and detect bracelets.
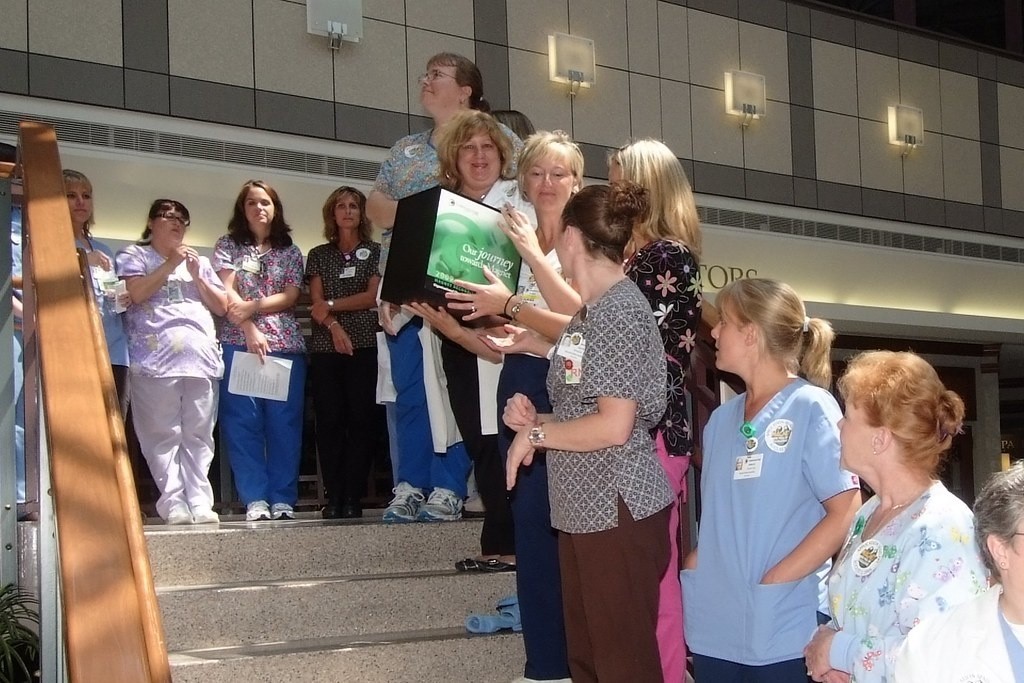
[504,294,516,315]
[254,298,260,314]
[328,321,338,329]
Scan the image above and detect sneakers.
[382,482,424,522]
[247,499,271,521]
[414,486,463,522]
[270,502,297,520]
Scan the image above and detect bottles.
[97,266,118,313]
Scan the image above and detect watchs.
[512,301,527,320]
[529,422,545,449]
[328,299,335,312]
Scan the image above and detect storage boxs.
[381,187,523,326]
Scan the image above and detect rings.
[808,669,814,675]
[511,224,517,228]
[471,302,476,312]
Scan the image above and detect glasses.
[154,213,191,228]
[417,68,458,84]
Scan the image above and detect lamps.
[547,32,596,97]
[724,69,766,129]
[306,0,362,50]
[888,104,924,158]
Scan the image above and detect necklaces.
[860,491,918,543]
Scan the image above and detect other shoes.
[322,500,342,518]
[478,558,517,572]
[166,510,195,526]
[196,509,220,523]
[343,501,363,518]
[455,557,481,571]
[513,674,572,683]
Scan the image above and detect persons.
[439,111,585,572]
[210,179,303,523]
[503,184,674,683]
[681,279,861,683]
[607,140,704,571]
[305,52,527,520]
[804,348,965,683]
[116,198,228,522]
[735,458,743,470]
[0,146,132,521]
[893,457,1024,683]
